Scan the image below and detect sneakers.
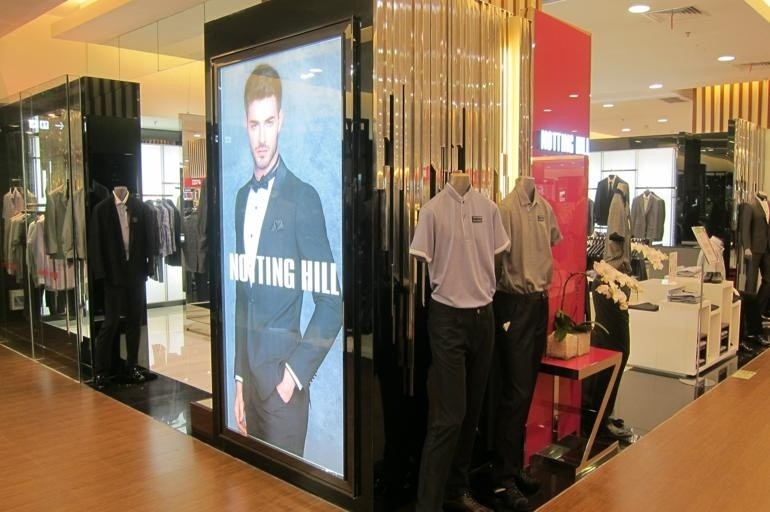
[702,272,723,283]
[599,416,632,441]
[485,471,543,511]
[443,491,492,512]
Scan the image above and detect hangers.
[587,235,642,245]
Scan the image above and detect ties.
[608,182,612,196]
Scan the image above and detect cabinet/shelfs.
[627,274,742,378]
[538,348,625,482]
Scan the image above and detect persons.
[408,172,510,511]
[696,236,770,354]
[603,182,632,277]
[495,176,563,510]
[592,270,631,441]
[89,186,156,385]
[740,191,770,320]
[233,66,345,460]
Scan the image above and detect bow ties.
[761,198,767,201]
[251,174,268,193]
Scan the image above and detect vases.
[546,325,592,360]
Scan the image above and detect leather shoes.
[739,341,754,354]
[745,334,770,347]
[123,365,158,382]
[94,374,112,393]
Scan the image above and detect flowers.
[552,260,644,342]
[632,241,668,271]
